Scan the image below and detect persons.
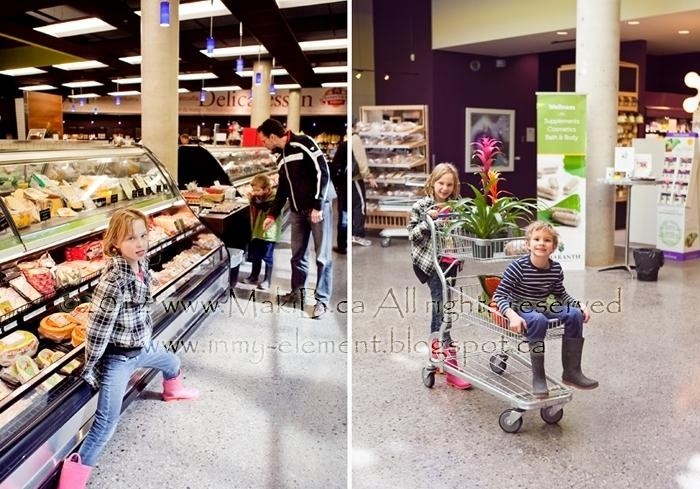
[352,117,378,246]
[406,162,472,390]
[57,209,199,489]
[241,175,284,289]
[329,141,347,255]
[256,119,337,319]
[492,220,598,400]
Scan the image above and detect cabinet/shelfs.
[1,142,230,489]
[178,144,291,233]
[359,105,429,230]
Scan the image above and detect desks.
[189,198,252,244]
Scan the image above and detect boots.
[431,337,472,389]
[161,368,198,401]
[561,334,599,390]
[529,340,549,399]
[58,458,93,489]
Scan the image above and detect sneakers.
[352,236,372,246]
[258,280,271,289]
[241,275,260,284]
[281,289,305,305]
[313,303,324,316]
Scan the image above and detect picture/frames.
[465,106,516,174]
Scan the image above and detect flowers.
[487,168,552,232]
[424,137,521,240]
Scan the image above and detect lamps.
[71,77,121,115]
[160,0,276,101]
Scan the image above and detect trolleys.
[414,203,590,437]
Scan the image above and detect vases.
[492,232,508,252]
[472,240,493,258]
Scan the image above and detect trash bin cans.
[634,248,664,281]
[227,248,244,288]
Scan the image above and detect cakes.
[1,303,92,385]
